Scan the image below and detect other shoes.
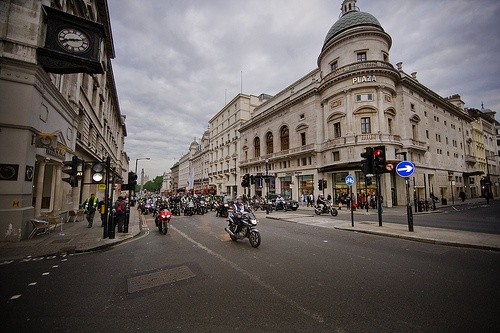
[88,223,92,227]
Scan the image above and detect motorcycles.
[154,209,172,235]
[315,199,338,216]
[225,209,262,248]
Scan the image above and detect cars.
[267,194,300,210]
[139,193,270,216]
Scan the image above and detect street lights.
[486,154,500,199]
[134,157,150,203]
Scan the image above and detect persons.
[301,193,313,207]
[317,194,328,211]
[138,191,229,227]
[338,191,384,214]
[98,196,105,227]
[459,190,466,202]
[241,194,285,211]
[108,194,138,233]
[232,195,244,234]
[82,193,99,228]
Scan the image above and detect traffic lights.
[62,156,78,187]
[240,173,250,187]
[373,146,386,173]
[121,184,128,190]
[128,172,137,189]
[360,147,373,174]
